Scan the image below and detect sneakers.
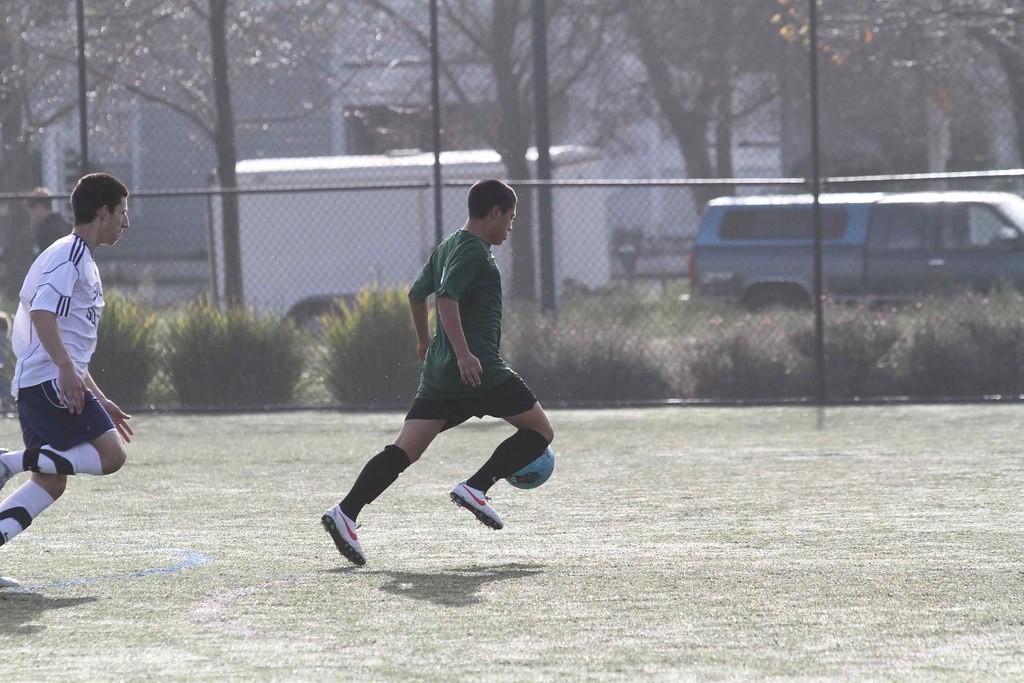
[450,479,503,531]
[320,504,366,565]
[0,449,15,492]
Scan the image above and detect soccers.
[506,445,555,490]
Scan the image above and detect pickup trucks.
[691,192,1024,318]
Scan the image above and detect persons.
[0,173,134,586]
[0,189,74,415]
[319,180,555,565]
